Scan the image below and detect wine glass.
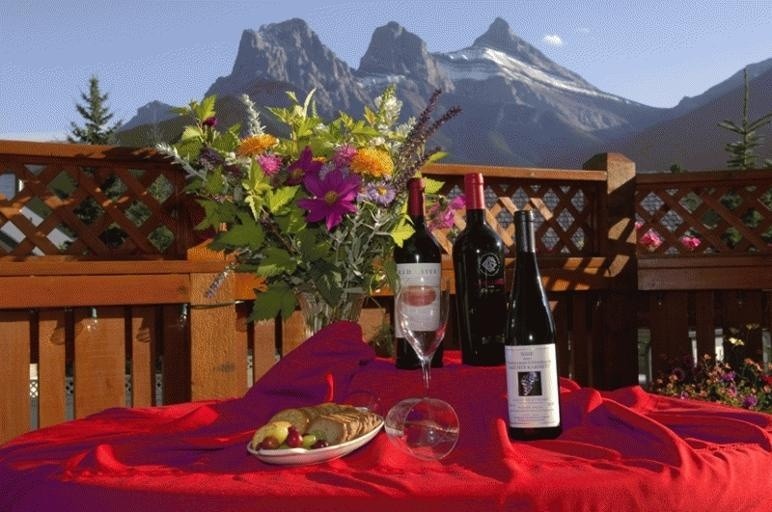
[341,388,461,462]
[395,276,450,399]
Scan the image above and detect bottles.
[393,177,445,370]
[451,173,507,368]
[504,210,563,440]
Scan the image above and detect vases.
[295,280,368,342]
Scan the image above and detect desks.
[4,386,772,512]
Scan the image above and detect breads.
[253,401,381,452]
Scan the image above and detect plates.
[247,407,385,467]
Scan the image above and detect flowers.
[172,84,466,321]
[644,323,772,414]
